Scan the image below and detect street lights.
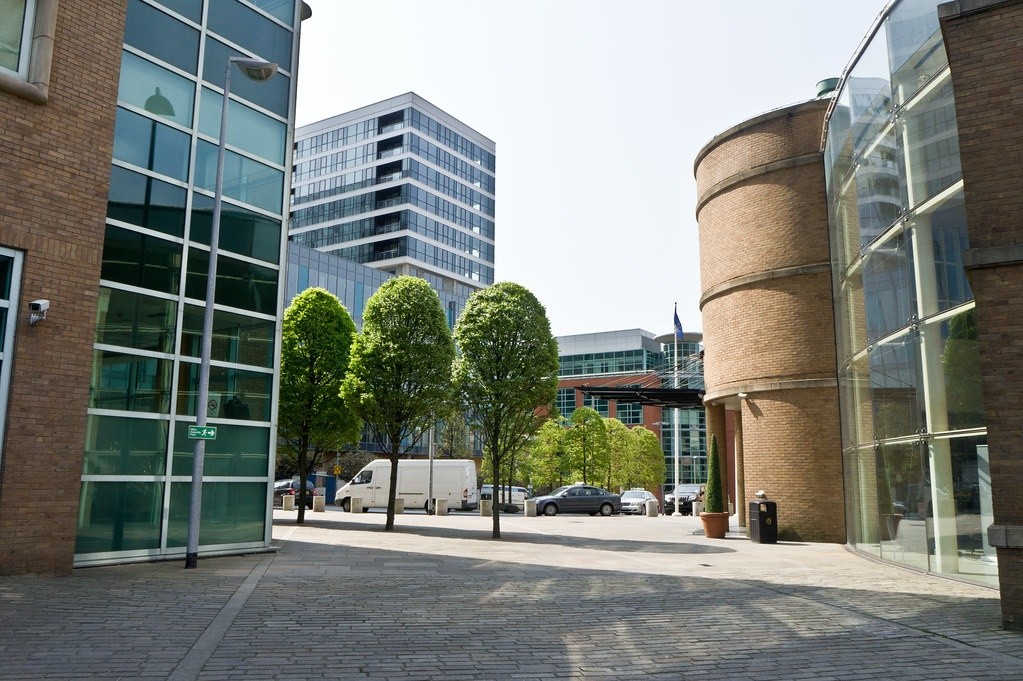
[112,87,176,550]
[186,56,278,568]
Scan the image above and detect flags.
[674,310,684,340]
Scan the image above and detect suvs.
[664,484,708,516]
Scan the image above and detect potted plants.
[700,434,730,539]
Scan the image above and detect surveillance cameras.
[738,393,748,399]
[28,300,50,312]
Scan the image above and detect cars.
[620,488,660,515]
[273,478,318,509]
[526,482,622,516]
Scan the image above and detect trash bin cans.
[749,500,778,544]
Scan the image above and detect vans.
[335,459,477,515]
[481,484,533,501]
